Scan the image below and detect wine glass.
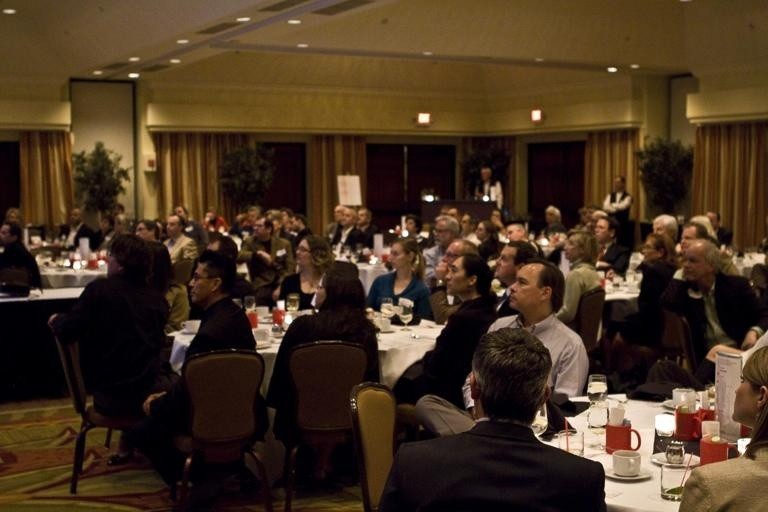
[231,292,413,331]
[589,404,608,452]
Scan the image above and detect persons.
[474,165,505,210]
[61,206,312,305]
[614,211,767,387]
[49,233,380,496]
[603,176,633,226]
[680,346,767,511]
[331,206,375,252]
[1,209,42,299]
[370,205,628,436]
[379,328,607,511]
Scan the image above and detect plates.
[603,465,651,480]
[256,342,274,349]
[650,451,700,467]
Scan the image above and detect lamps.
[417,112,431,126]
[527,107,545,125]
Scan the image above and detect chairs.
[348,381,398,508]
[46,310,154,495]
[174,347,273,511]
[6,206,766,401]
[285,342,367,511]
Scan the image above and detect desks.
[1,204,767,512]
[542,398,751,511]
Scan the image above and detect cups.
[340,242,363,266]
[661,462,691,501]
[586,372,607,404]
[651,385,721,442]
[666,443,684,463]
[180,320,201,333]
[597,270,640,293]
[606,423,642,454]
[700,438,739,467]
[252,328,270,343]
[559,430,585,456]
[611,448,642,476]
[39,249,107,273]
